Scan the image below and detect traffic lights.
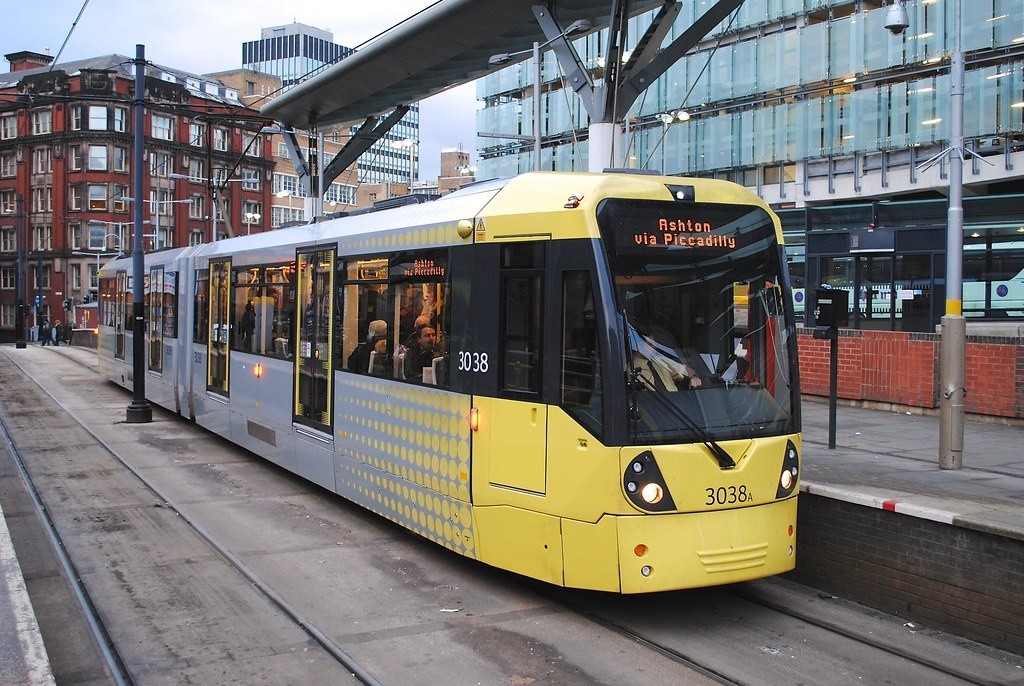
[90,293,97,304]
[63,297,74,311]
[23,304,32,316]
[83,293,92,304]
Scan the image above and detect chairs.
[432,357,443,385]
[369,351,407,380]
[275,338,286,357]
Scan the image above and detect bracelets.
[689,375,699,380]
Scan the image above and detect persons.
[41,320,56,346]
[54,320,68,346]
[239,279,341,356]
[347,282,446,382]
[627,332,703,393]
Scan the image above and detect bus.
[97,170,805,591]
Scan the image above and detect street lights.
[170,173,259,241]
[260,127,354,214]
[245,211,262,236]
[273,190,293,222]
[488,19,594,173]
[119,195,194,248]
[90,220,151,252]
[885,0,968,470]
[72,251,123,270]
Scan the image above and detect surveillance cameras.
[884,4,909,36]
